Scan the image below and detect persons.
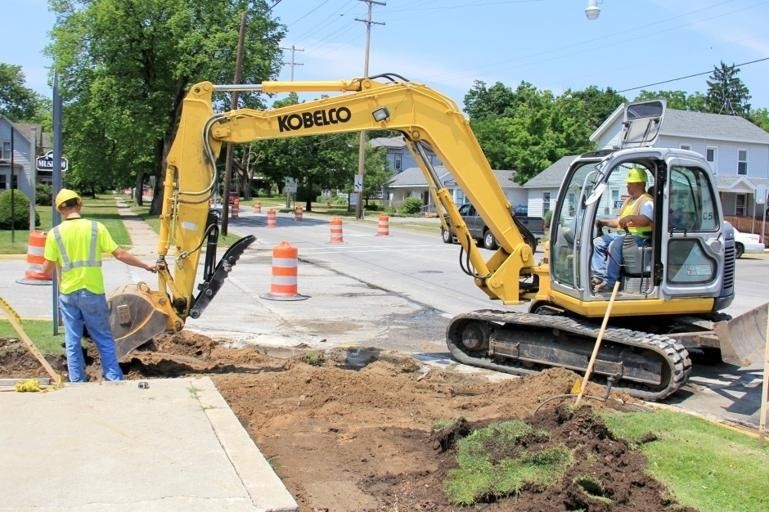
[591,167,654,293]
[43,188,165,382]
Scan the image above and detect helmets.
[55,188,81,210]
[624,167,648,184]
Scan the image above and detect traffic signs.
[35,150,69,172]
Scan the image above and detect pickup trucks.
[434,197,548,250]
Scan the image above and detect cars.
[724,219,766,259]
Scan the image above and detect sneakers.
[591,277,609,291]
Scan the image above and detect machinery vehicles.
[106,65,769,407]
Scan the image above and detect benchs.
[623,210,700,295]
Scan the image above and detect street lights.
[584,0,603,22]
